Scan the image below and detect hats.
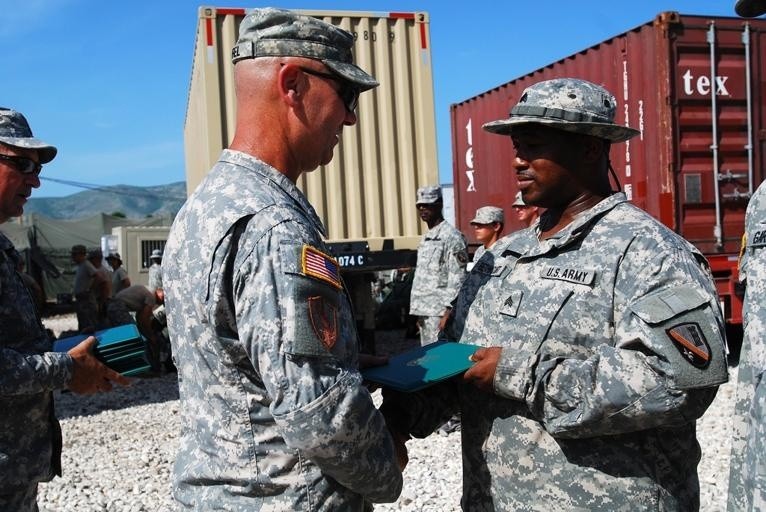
[483,77,640,142]
[68,243,123,265]
[0,108,56,164]
[512,192,527,206]
[148,250,162,258]
[469,206,504,226]
[416,185,441,205]
[231,8,380,92]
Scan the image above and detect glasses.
[280,60,360,112]
[0,157,45,175]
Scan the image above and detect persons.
[407,185,468,349]
[510,190,540,230]
[730,176,765,512]
[161,5,410,512]
[468,203,504,266]
[17,253,42,302]
[68,245,177,374]
[0,107,131,509]
[382,78,729,511]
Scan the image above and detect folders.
[360,340,486,395]
[49,322,154,394]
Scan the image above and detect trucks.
[172,6,444,350]
[440,12,766,354]
